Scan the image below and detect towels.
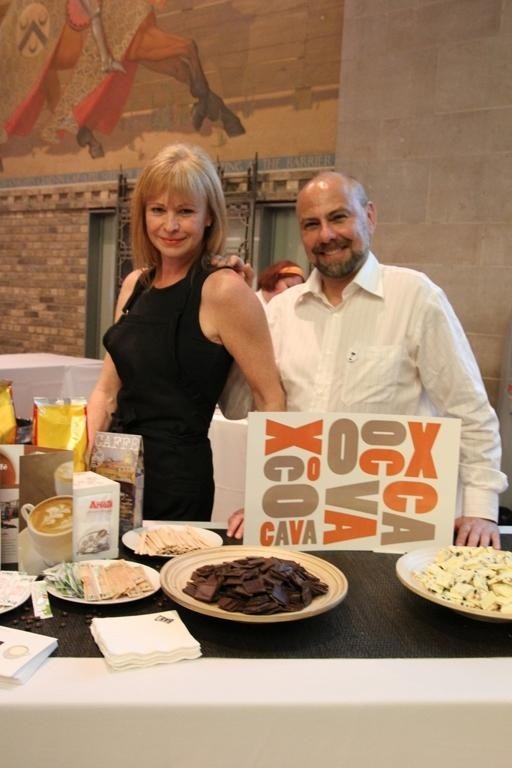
[89,610,203,672]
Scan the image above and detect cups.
[20,494,73,565]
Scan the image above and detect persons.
[210,170,509,552]
[254,259,306,306]
[84,142,287,541]
[81,0,128,74]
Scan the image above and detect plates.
[395,543,511,623]
[43,557,160,605]
[121,523,224,557]
[159,543,348,624]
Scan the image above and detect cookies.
[412,544,512,612]
[182,556,328,615]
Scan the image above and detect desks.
[0,526,512,768]
